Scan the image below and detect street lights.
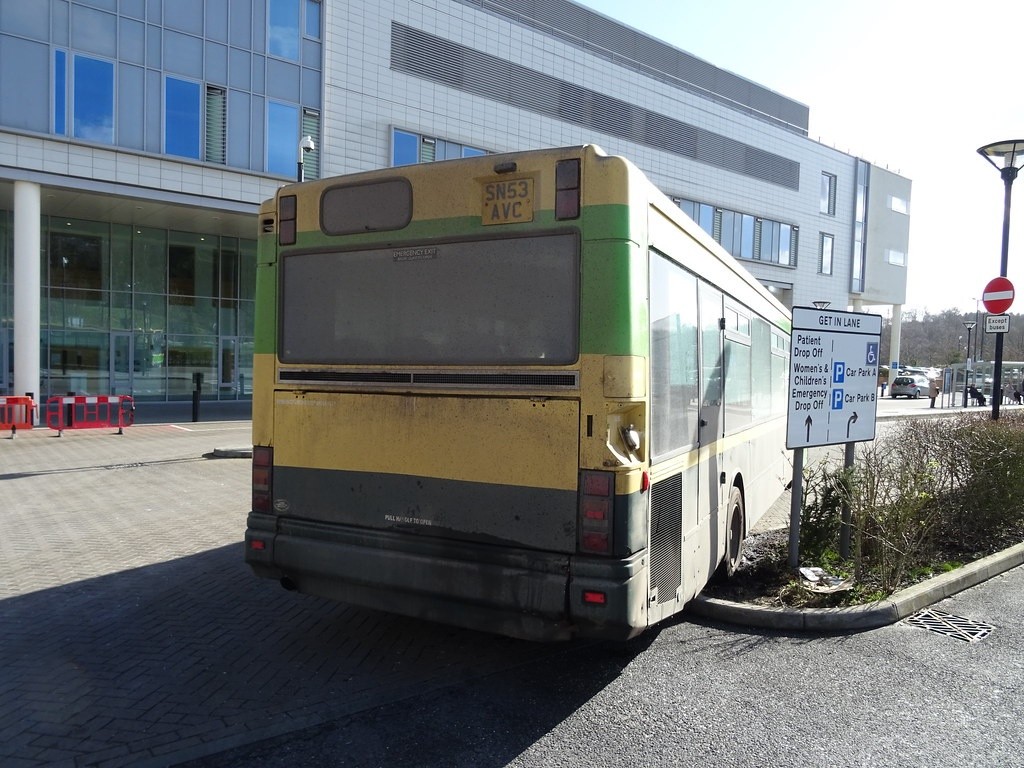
[962,321,978,408]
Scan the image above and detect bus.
[243,141,825,641]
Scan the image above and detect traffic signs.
[786,305,882,450]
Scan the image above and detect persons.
[928,378,940,409]
[969,383,986,407]
[1005,380,1023,405]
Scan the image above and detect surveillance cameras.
[302,140,314,153]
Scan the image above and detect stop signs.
[982,277,1015,315]
